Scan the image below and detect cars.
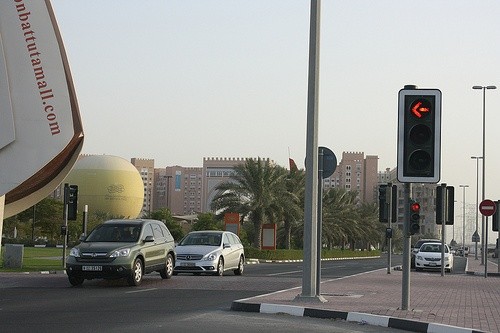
[414,242,455,273]
[172,230,246,277]
[451,248,456,255]
[455,249,464,256]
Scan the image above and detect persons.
[127,228,140,242]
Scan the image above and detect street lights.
[458,184,469,257]
[470,156,483,260]
[471,85,497,264]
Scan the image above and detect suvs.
[411,238,441,269]
[65,218,178,286]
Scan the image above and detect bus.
[480,247,497,258]
[460,246,469,255]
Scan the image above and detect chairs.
[426,247,432,251]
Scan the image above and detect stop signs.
[478,199,498,217]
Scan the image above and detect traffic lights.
[396,87,443,184]
[69,186,77,204]
[410,202,420,234]
